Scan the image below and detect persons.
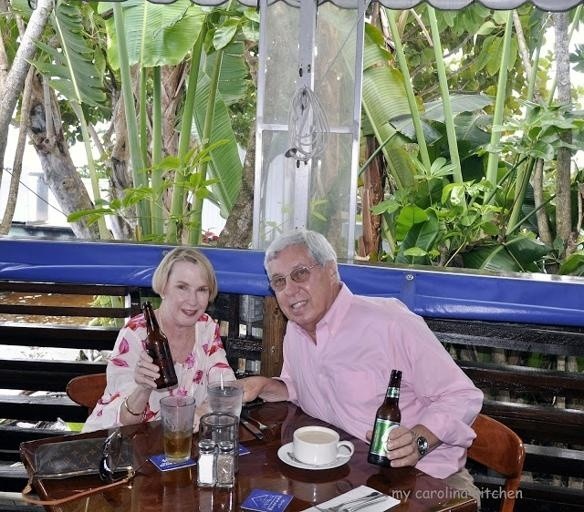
[75,244,235,437]
[230,228,487,509]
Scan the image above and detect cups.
[289,480,353,503]
[289,421,354,465]
[156,383,251,471]
[158,466,195,511]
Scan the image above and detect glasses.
[98,428,123,483]
[268,264,317,292]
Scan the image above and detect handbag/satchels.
[20,435,133,506]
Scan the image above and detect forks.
[315,492,387,511]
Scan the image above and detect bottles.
[366,370,405,468]
[195,439,235,491]
[197,490,234,512]
[141,300,179,390]
[367,471,391,496]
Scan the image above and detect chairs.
[65,373,107,415]
[465,413,527,511]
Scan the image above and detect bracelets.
[122,399,149,417]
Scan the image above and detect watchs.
[410,430,428,463]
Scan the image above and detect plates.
[277,441,355,474]
[276,462,351,483]
